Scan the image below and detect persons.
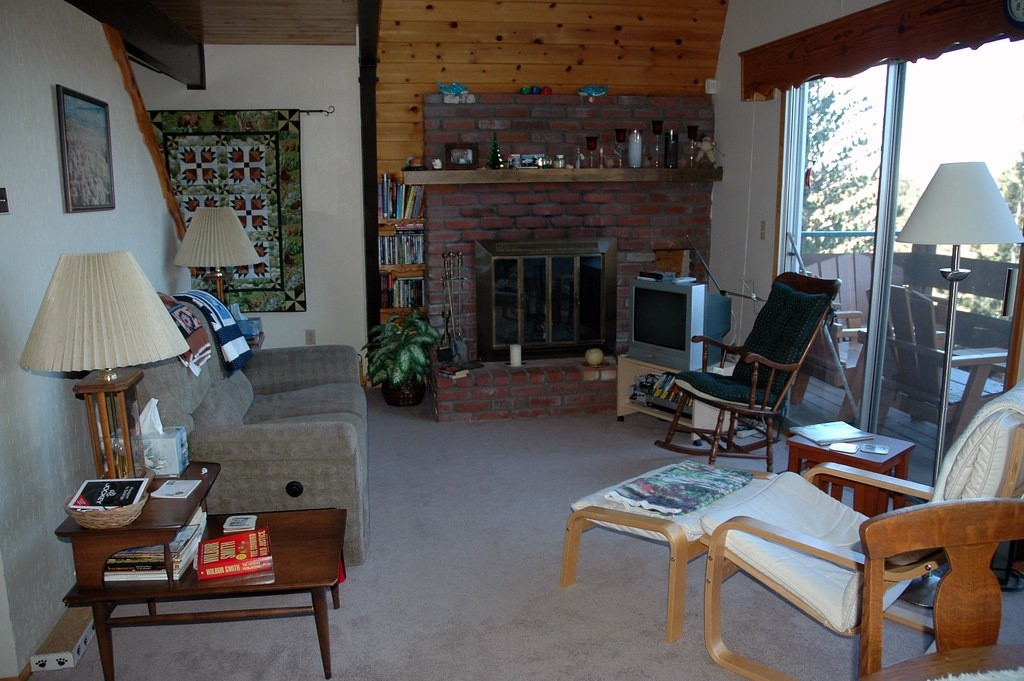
[692,134,719,169]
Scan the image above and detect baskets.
[62,490,149,531]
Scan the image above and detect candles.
[627,128,642,168]
[510,344,522,366]
[665,127,679,170]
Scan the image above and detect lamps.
[21,248,190,483]
[895,161,1024,487]
[173,206,263,302]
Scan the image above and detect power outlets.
[305,329,315,345]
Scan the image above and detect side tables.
[52,461,347,681]
[786,431,916,514]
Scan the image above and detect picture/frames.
[56,84,116,214]
[445,142,479,170]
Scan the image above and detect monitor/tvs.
[628,278,732,372]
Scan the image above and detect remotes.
[671,276,696,283]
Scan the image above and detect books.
[378,223,424,266]
[378,173,425,220]
[104,506,274,580]
[379,272,423,310]
[151,480,202,498]
[436,365,470,379]
[789,421,875,446]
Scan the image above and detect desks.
[860,643,1024,681]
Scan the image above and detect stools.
[560,463,778,643]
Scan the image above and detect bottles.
[554,155,565,168]
[629,128,643,168]
[664,128,679,168]
[409,156,422,166]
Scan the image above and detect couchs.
[105,288,370,566]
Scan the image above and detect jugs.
[432,159,442,169]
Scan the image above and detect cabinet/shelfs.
[616,354,739,445]
[380,218,427,324]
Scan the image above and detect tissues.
[105,398,191,480]
[230,303,263,336]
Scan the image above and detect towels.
[171,288,256,380]
[155,291,212,379]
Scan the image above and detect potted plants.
[360,301,442,407]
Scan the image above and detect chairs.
[653,270,842,474]
[697,379,1024,681]
[791,253,1009,453]
